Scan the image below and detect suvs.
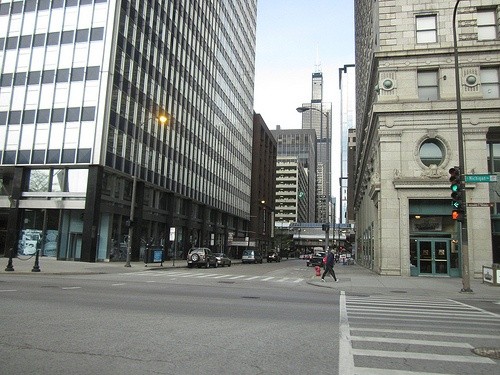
[187,248,218,268]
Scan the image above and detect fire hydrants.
[315,266,321,276]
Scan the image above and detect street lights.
[125,116,169,266]
[296,107,331,247]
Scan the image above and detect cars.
[214,252,231,266]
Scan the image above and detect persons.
[321,248,340,282]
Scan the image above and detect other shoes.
[336,279,339,283]
[320,279,327,282]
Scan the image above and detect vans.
[242,250,263,264]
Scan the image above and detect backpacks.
[323,256,329,265]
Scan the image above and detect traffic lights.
[448,167,464,221]
[322,223,325,230]
